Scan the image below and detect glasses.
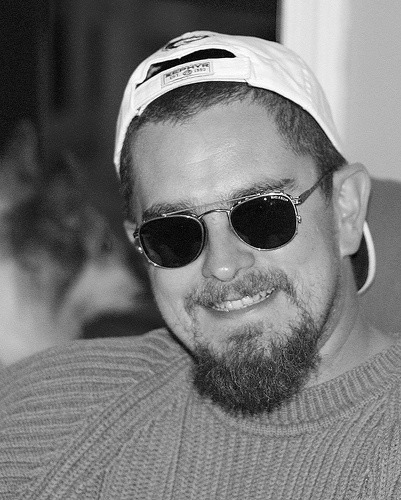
[133,165,338,270]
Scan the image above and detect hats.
[113,29,378,297]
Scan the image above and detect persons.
[0,28,401,499]
